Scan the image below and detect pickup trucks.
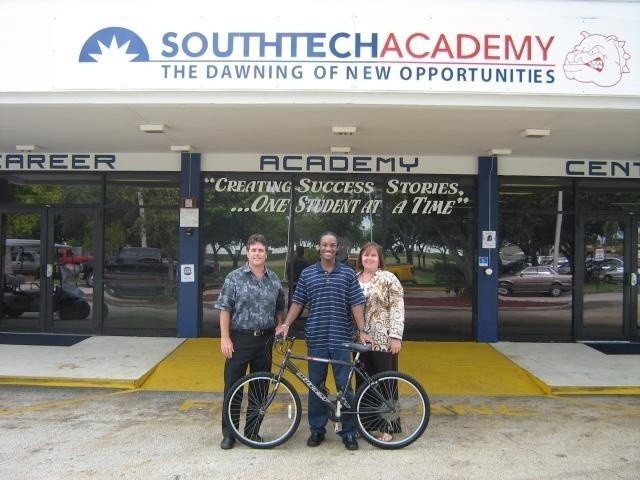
[344,257,417,285]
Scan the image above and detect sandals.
[377,433,393,441]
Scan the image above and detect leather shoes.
[307,431,325,447]
[244,434,264,443]
[343,436,358,449]
[220,437,237,449]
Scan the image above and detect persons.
[352,242,405,442]
[274,231,376,450]
[286,246,309,320]
[212,234,285,449]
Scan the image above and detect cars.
[4,238,95,278]
[499,252,624,281]
[169,255,220,280]
[498,265,572,297]
[86,247,177,308]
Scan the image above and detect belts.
[231,329,276,337]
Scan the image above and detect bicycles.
[224,335,430,450]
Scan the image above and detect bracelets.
[282,324,290,328]
[359,328,364,331]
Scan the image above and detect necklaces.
[362,273,375,291]
